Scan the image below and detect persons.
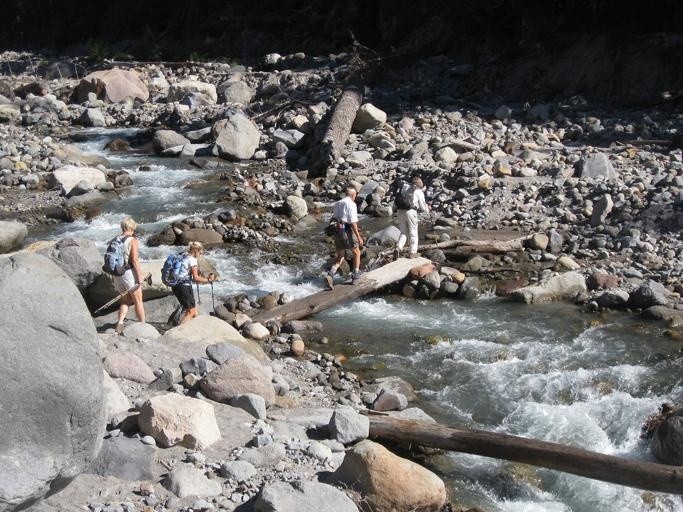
[173,242,214,325]
[324,187,363,289]
[392,177,431,261]
[106,218,145,333]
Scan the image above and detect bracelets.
[208,279,210,283]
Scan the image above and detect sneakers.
[408,253,421,258]
[325,275,334,290]
[352,275,365,285]
[393,248,399,261]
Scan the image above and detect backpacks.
[395,181,417,210]
[102,235,132,277]
[161,252,190,287]
[325,218,351,240]
